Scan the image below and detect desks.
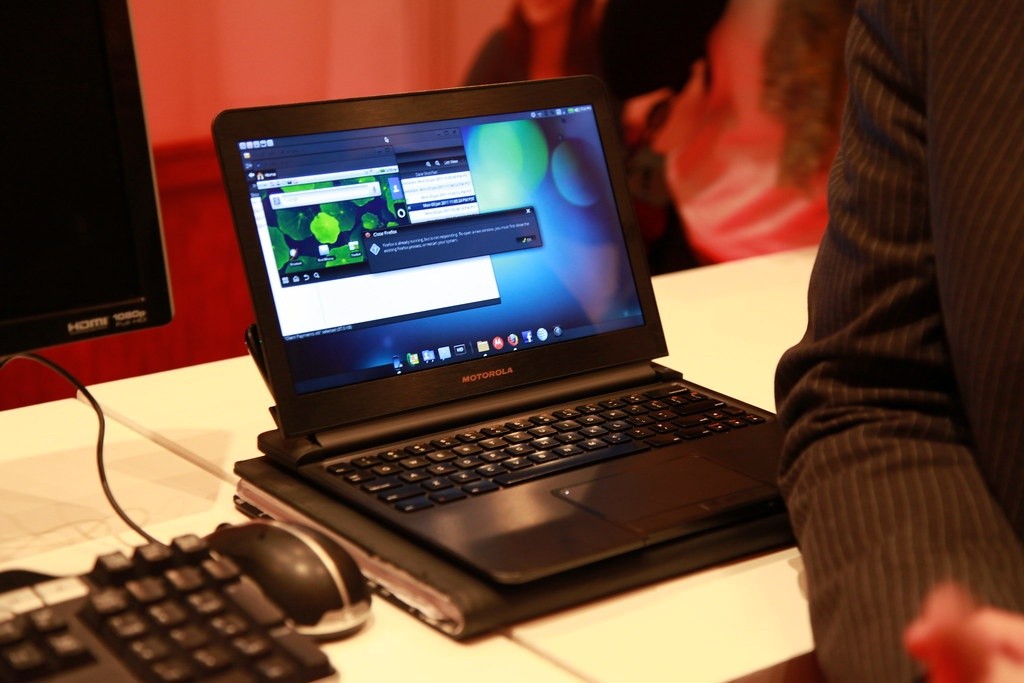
[76,244,818,683]
[0,398,595,683]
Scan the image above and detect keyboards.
[0,534,340,683]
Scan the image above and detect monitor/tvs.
[0,0,176,356]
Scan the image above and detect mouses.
[208,521,372,635]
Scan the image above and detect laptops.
[212,75,786,587]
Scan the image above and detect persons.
[596,2,855,281]
[771,0,1024,683]
[457,0,604,90]
